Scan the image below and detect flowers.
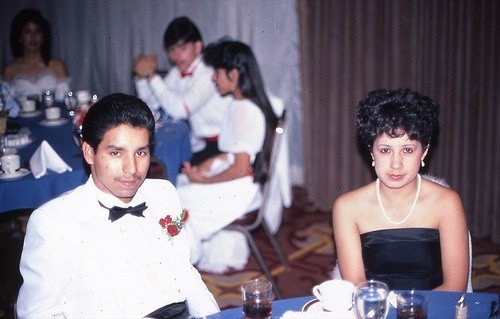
[159,209,189,242]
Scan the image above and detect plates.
[0,169,31,180]
[0,134,37,148]
[17,111,69,126]
[301,298,355,312]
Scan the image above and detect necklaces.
[376,174,422,224]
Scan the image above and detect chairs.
[145,108,290,301]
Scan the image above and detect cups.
[312,280,356,312]
[44,95,54,107]
[397,292,428,319]
[45,107,61,121]
[65,96,76,109]
[21,99,36,112]
[351,279,390,319]
[241,278,273,319]
[0,155,21,173]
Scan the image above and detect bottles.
[455,296,467,319]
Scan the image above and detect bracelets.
[147,70,158,77]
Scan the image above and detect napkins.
[29,139,74,179]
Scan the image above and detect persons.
[16,93,220,319]
[0,8,68,101]
[332,88,469,291]
[177,42,279,246]
[136,16,218,162]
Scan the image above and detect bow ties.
[98,200,148,222]
[178,66,197,78]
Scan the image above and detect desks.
[199,289,500,319]
[0,103,193,214]
